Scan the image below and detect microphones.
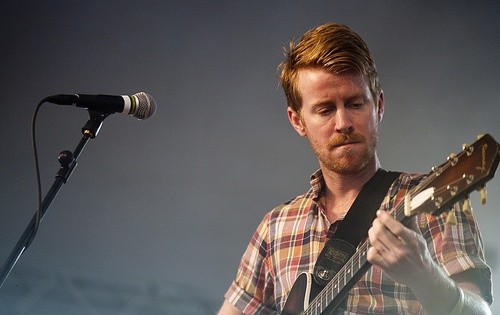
[44,90,157,120]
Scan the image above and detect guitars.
[281,132,500,315]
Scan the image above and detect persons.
[217,19,494,315]
